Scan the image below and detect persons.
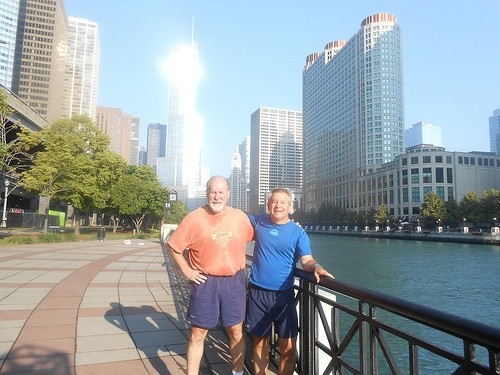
[168,176,301,375]
[199,187,335,375]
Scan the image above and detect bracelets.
[312,264,323,273]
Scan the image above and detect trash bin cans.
[96,227,106,241]
